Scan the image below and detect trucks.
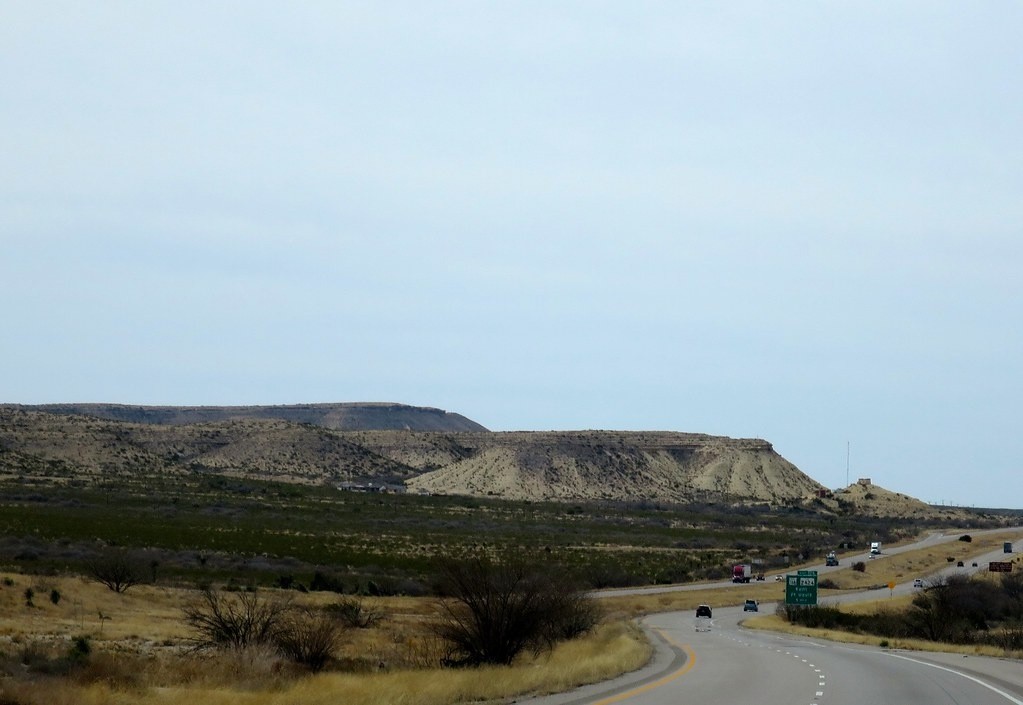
[826,556,839,566]
[871,542,882,553]
[1004,541,1012,553]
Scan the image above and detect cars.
[957,561,964,566]
[914,579,923,587]
[869,555,875,559]
[744,599,759,612]
[775,575,783,582]
[696,605,713,618]
[756,573,765,580]
[972,563,978,567]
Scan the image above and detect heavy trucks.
[732,565,752,583]
